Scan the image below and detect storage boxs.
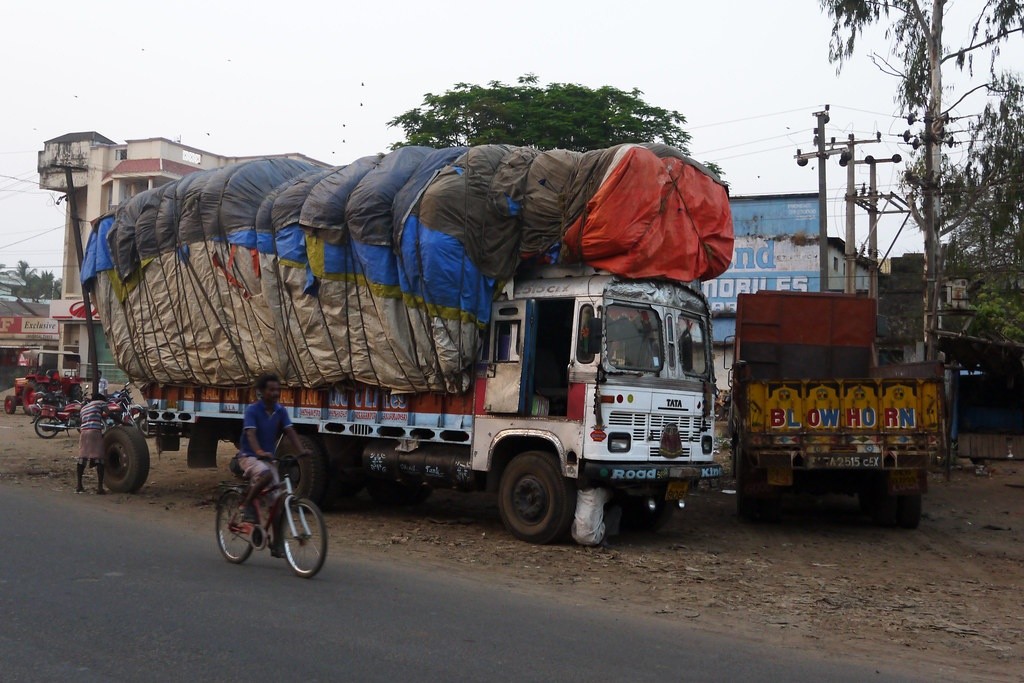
[41,405,57,417]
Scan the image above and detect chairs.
[533,349,568,400]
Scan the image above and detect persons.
[76,394,133,495]
[239,375,310,559]
[98,371,108,395]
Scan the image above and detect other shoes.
[270,549,288,558]
[229,521,253,534]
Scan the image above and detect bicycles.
[214,454,327,579]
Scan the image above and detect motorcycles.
[5,350,155,438]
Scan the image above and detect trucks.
[144,264,723,545]
[724,290,944,529]
